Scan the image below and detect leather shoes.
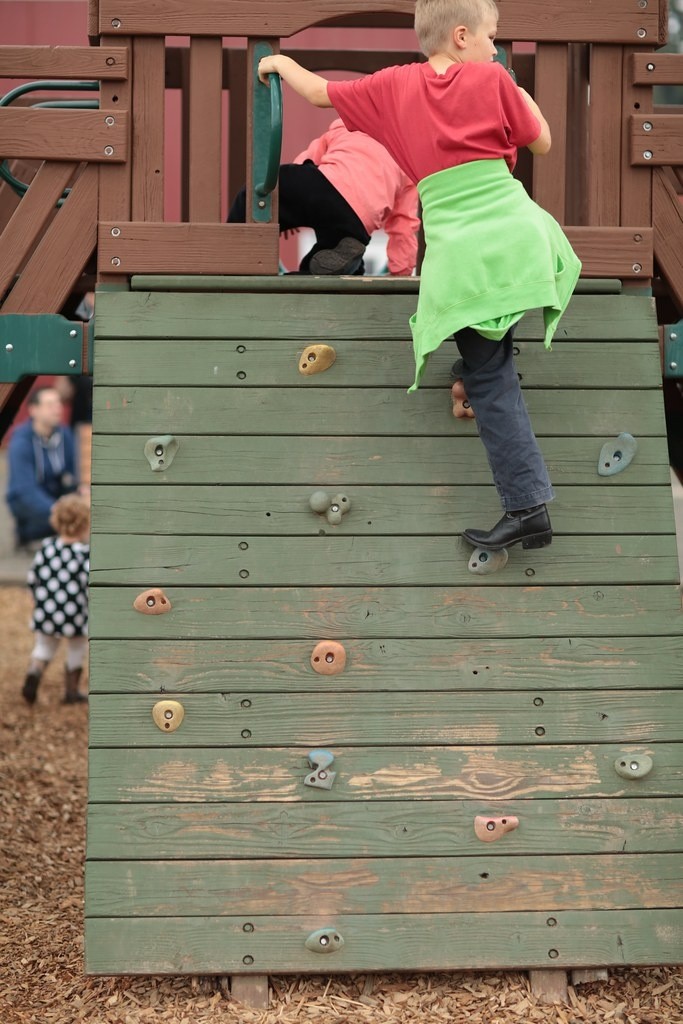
[450,357,464,379]
[461,504,553,551]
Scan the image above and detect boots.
[65,663,87,704]
[22,657,49,704]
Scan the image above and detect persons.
[226,117,420,275]
[5,387,79,548]
[21,493,90,705]
[257,0,581,550]
[54,276,95,430]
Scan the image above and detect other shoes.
[309,237,366,275]
